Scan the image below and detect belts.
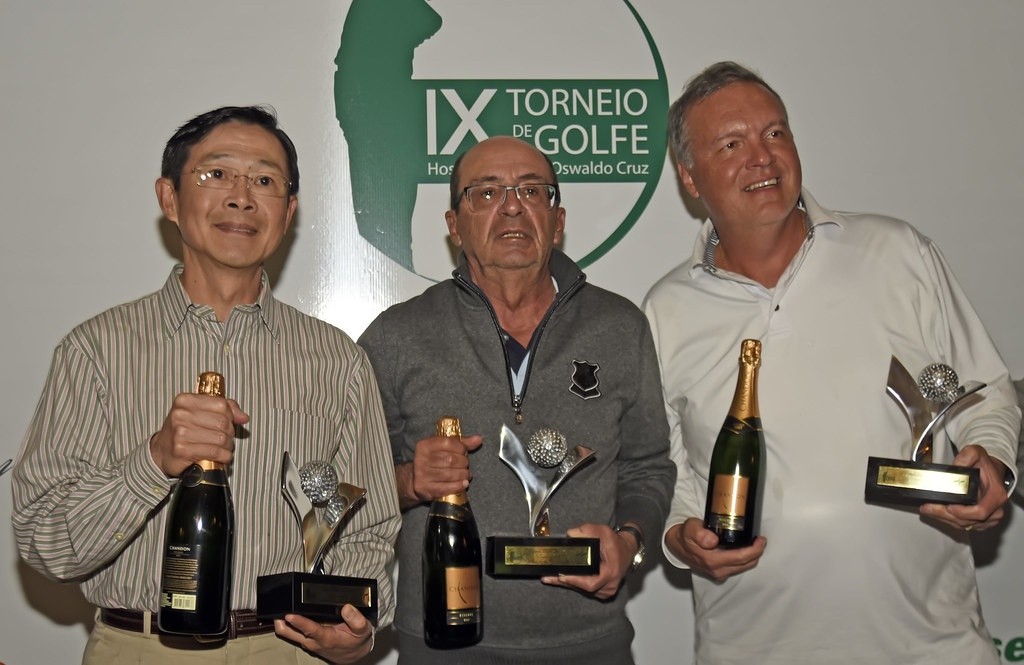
[101,605,284,645]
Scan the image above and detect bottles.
[423,417,484,650]
[157,371,234,638]
[702,339,762,550]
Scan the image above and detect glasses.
[178,163,291,199]
[453,182,557,212]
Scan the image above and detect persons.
[640,61,1024,665]
[356,134,678,665]
[10,107,405,665]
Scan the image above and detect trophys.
[255,451,379,625]
[485,422,601,580]
[865,354,987,507]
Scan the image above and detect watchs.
[613,524,648,575]
